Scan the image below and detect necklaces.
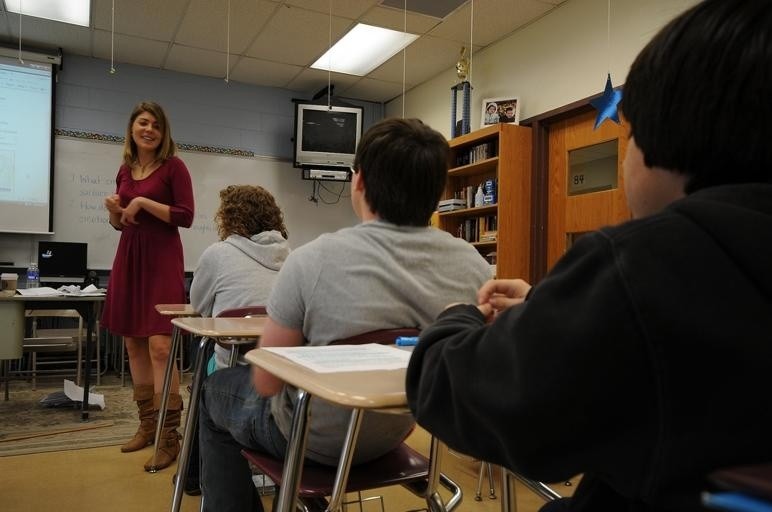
[137,158,153,177]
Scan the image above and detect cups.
[0,273,20,297]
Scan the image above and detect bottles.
[474,186,485,208]
[26,263,42,289]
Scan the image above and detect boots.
[144,392,183,471]
[121,383,154,453]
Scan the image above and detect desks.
[0,286,106,422]
[244,343,420,512]
[155,304,199,317]
[170,317,270,512]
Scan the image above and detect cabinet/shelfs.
[430,122,532,288]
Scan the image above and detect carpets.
[0,372,193,457]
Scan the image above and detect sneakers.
[173,473,201,495]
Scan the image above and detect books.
[453,216,497,244]
[453,185,475,208]
[469,142,490,163]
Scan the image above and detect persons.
[98,101,194,472]
[404,0,772,512]
[485,102,516,125]
[173,184,292,495]
[199,120,496,512]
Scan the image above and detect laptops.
[38,241,87,284]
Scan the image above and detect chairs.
[215,306,266,368]
[22,309,84,390]
[240,329,462,512]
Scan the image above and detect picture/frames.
[481,95,520,126]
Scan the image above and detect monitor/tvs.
[296,104,362,167]
